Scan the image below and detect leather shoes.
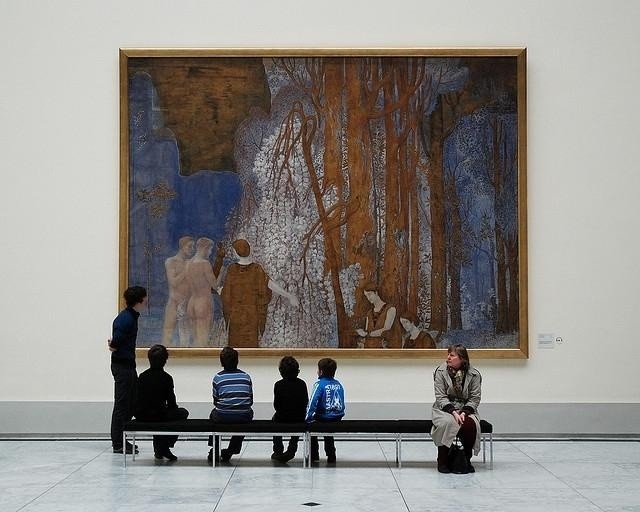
[437,458,450,473]
[468,461,475,473]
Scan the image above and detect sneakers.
[281,449,295,462]
[158,448,177,460]
[302,452,320,463]
[208,448,219,462]
[271,452,282,460]
[221,449,231,461]
[125,440,138,450]
[327,456,336,463]
[154,452,164,459]
[113,448,139,454]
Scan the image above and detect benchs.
[215,420,308,466]
[122,421,218,467]
[307,420,400,467]
[399,420,492,466]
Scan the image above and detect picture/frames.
[119,48,528,359]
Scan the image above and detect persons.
[108,286,148,454]
[207,347,254,463]
[304,358,345,464]
[270,356,309,462]
[400,312,437,349]
[186,238,224,347]
[133,344,189,461]
[356,283,402,348]
[429,343,481,474]
[162,236,195,347]
[217,239,299,348]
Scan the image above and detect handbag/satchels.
[447,443,470,474]
